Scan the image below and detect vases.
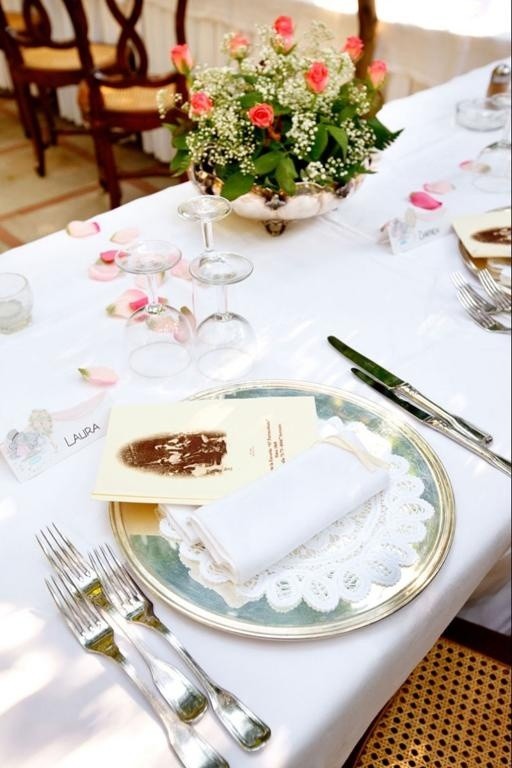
[181,157,372,235]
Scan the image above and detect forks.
[44,567,231,768]
[88,544,270,751]
[447,240,511,335]
[35,521,210,725]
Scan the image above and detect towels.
[190,432,390,582]
[157,503,208,547]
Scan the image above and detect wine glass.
[114,239,193,376]
[187,251,260,379]
[177,195,241,321]
[469,93,511,194]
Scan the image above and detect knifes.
[350,367,511,476]
[328,333,491,444]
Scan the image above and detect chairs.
[62,0,188,212]
[346,616,512,767]
[0,0,136,176]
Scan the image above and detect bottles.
[483,64,510,101]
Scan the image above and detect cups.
[1,271,35,334]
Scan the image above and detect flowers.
[155,15,404,205]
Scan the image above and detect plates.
[111,383,456,643]
[450,208,512,257]
